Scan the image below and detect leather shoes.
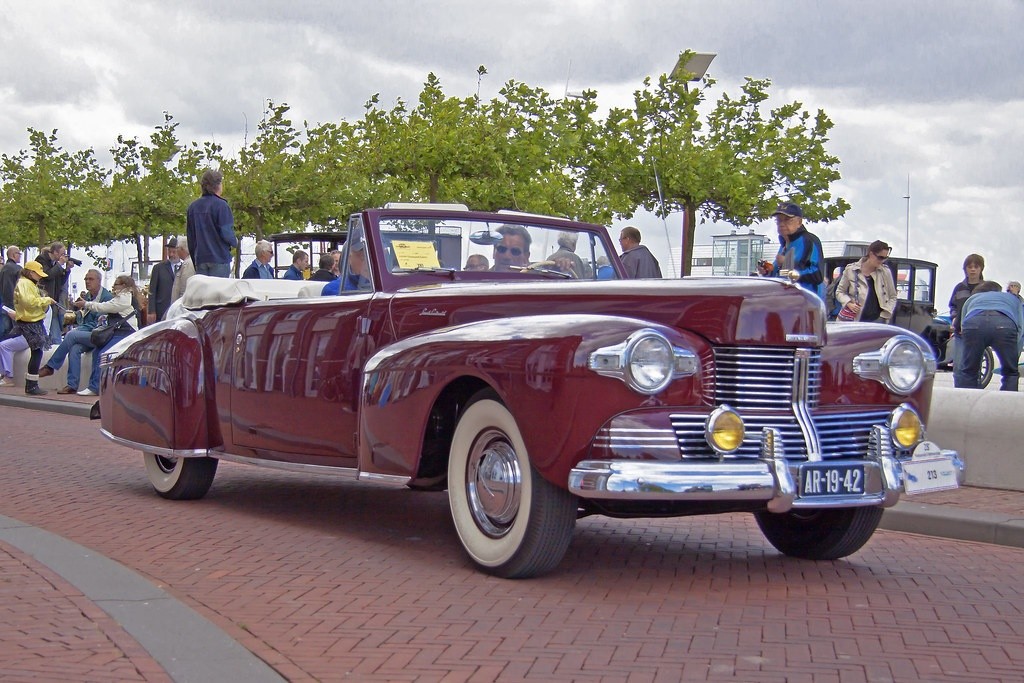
[38,365,54,377]
[58,385,76,394]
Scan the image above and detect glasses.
[266,250,273,254]
[15,251,22,255]
[873,252,889,261]
[774,217,795,225]
[84,277,99,282]
[495,245,527,256]
[114,283,122,287]
[463,264,483,271]
[619,237,626,243]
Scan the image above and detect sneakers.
[77,388,97,396]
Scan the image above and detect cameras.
[65,254,82,267]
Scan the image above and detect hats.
[25,260,49,277]
[164,237,177,247]
[772,203,802,218]
[344,238,366,252]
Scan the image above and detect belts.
[976,310,1004,315]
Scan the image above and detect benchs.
[181,273,330,305]
[12,345,102,390]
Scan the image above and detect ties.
[174,265,178,276]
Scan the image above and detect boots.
[25,373,48,395]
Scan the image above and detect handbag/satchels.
[90,322,117,348]
[835,262,859,322]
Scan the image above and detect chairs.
[297,282,328,297]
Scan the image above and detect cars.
[823,255,994,389]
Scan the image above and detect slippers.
[0,374,14,386]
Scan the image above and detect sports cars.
[88,202,968,581]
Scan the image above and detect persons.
[321,228,388,296]
[597,255,615,278]
[546,231,584,279]
[241,240,274,278]
[756,201,825,294]
[38,269,114,394]
[488,224,571,274]
[825,277,842,321]
[619,226,663,278]
[464,254,489,271]
[330,251,342,277]
[948,253,986,387]
[836,240,897,324]
[0,242,74,395]
[73,275,148,396]
[952,280,1024,391]
[307,254,338,282]
[187,169,238,278]
[284,251,309,280]
[148,237,183,324]
[171,236,197,305]
